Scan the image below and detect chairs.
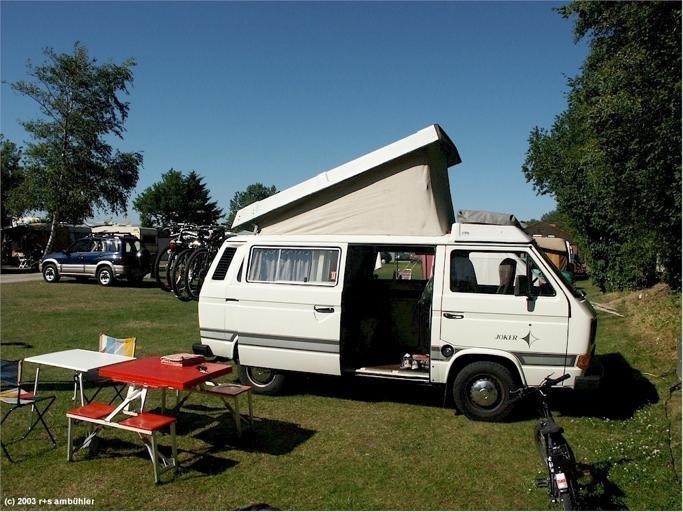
[495,258,516,294]
[1,334,137,464]
[17,252,33,268]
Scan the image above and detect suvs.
[36,231,150,289]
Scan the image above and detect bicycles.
[151,223,225,302]
[505,372,590,509]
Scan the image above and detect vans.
[192,123,613,423]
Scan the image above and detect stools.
[66,357,253,486]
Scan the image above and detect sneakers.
[412,361,418,370]
[399,360,412,370]
